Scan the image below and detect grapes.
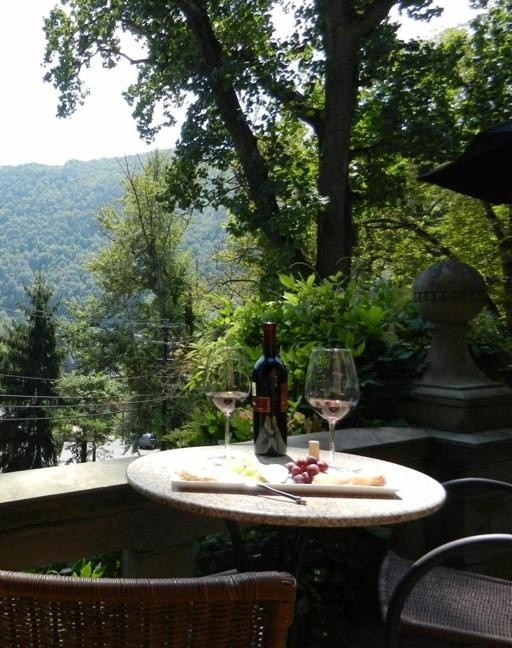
[286,456,328,483]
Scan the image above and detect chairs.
[378,477,511,648]
[0,570,298,648]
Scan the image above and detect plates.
[169,461,398,496]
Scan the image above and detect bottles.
[252,322,287,456]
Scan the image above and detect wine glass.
[305,349,359,464]
[201,346,250,464]
